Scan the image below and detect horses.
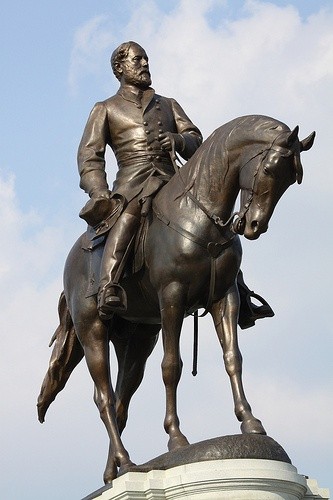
[35,115,317,485]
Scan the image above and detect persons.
[75,40,274,330]
